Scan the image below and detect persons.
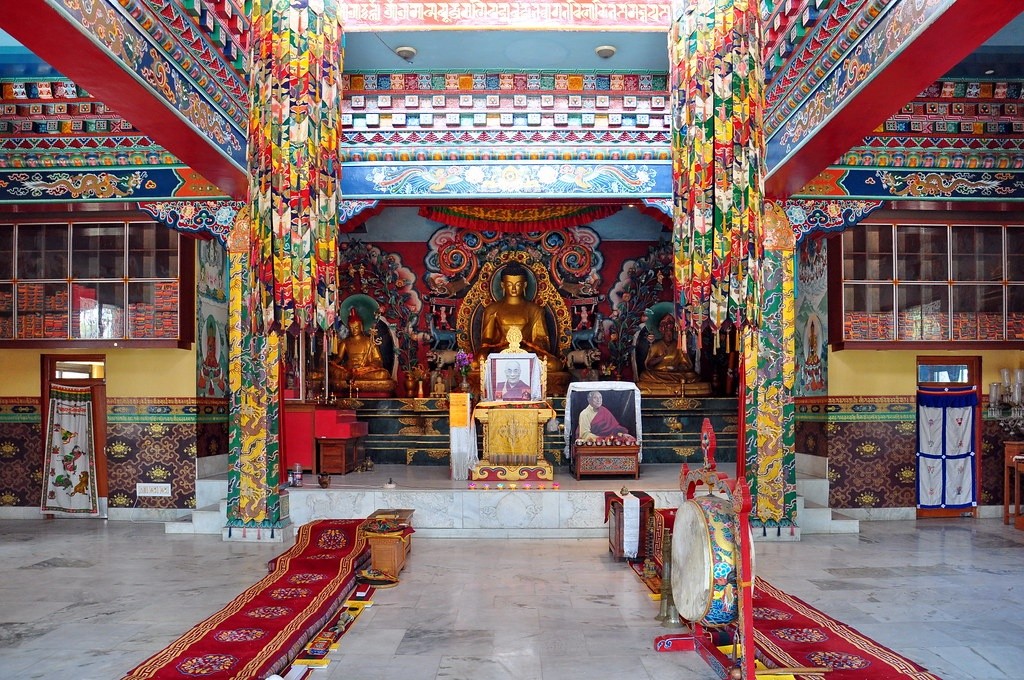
[497,364,529,399]
[641,316,699,383]
[434,375,445,392]
[329,307,389,380]
[469,262,562,370]
[577,392,634,441]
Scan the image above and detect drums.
[670,494,757,628]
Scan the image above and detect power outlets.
[136,483,172,497]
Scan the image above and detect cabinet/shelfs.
[605,491,654,562]
[0,201,198,351]
[318,439,366,475]
[826,218,1024,351]
[367,508,416,576]
[569,444,640,481]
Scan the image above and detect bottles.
[288,470,292,486]
[417,381,424,397]
[293,464,303,487]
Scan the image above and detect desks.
[1014,455,1024,531]
[1002,441,1024,525]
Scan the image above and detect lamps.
[988,367,1024,435]
